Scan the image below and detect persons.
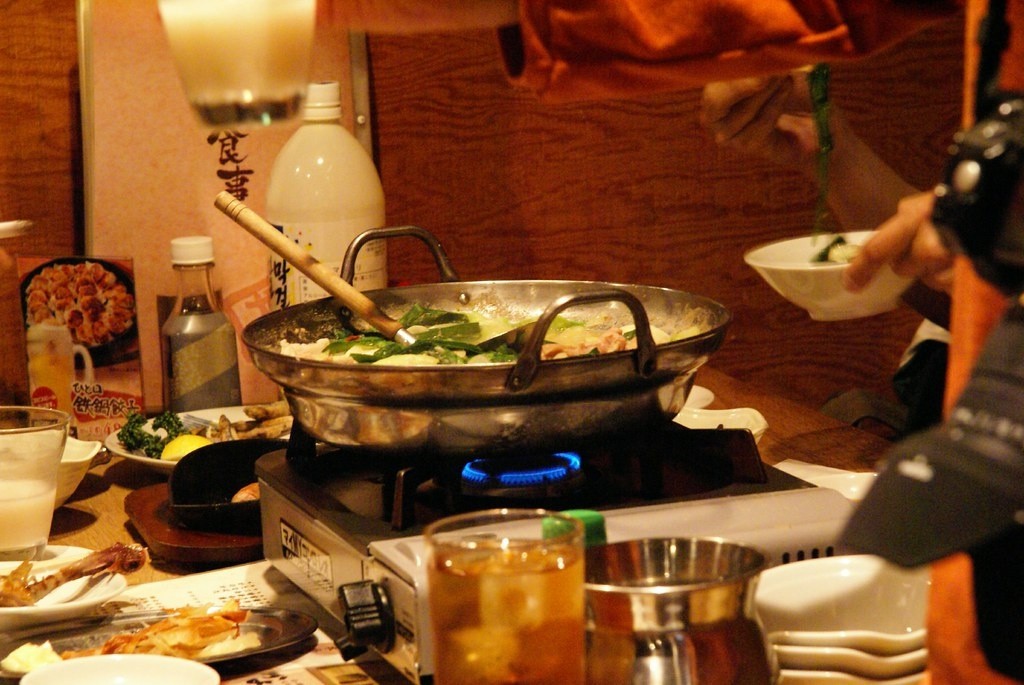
[316,1,1024,685]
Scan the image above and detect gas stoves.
[251,418,862,685]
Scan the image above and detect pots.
[240,225,730,450]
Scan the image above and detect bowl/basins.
[744,231,913,320]
[19,654,220,685]
[51,438,100,512]
[20,257,135,363]
[755,553,935,685]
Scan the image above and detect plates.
[168,435,288,529]
[0,608,318,678]
[104,406,263,475]
[0,545,127,631]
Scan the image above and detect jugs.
[581,536,778,684]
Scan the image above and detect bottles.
[0,221,35,418]
[266,82,387,307]
[540,511,604,644]
[160,237,242,414]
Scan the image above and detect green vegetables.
[116,410,187,458]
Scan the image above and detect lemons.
[160,433,214,461]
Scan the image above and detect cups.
[424,507,587,685]
[27,321,93,430]
[157,1,317,125]
[0,404,71,561]
